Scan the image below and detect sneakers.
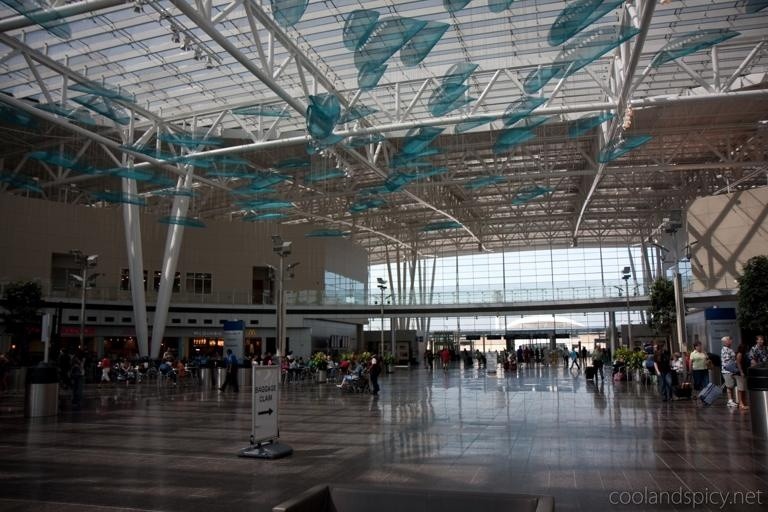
[336,384,342,388]
[727,401,739,407]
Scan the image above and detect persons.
[653,334,768,409]
[424,349,486,371]
[0,347,381,405]
[591,344,605,380]
[503,346,587,370]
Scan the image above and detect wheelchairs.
[345,370,370,394]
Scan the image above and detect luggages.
[697,381,725,406]
[586,367,594,379]
[681,374,692,389]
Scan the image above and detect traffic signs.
[251,364,281,442]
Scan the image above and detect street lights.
[374,277,392,359]
[613,267,643,352]
[265,235,300,359]
[69,249,106,377]
[642,217,699,382]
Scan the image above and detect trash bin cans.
[746,366,768,441]
[198,365,250,388]
[25,368,59,417]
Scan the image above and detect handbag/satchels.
[725,359,739,373]
